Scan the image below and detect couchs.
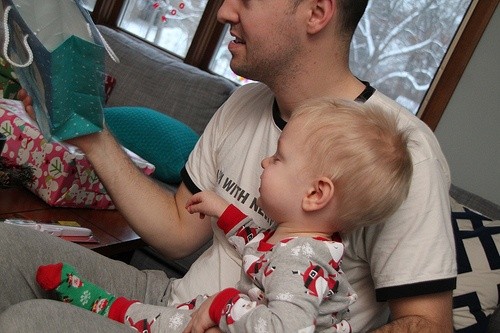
[97,25,500,333]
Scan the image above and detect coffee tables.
[0,189,150,266]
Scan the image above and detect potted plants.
[0,155,38,215]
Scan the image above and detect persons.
[36,98,412,333]
[18,0,457,333]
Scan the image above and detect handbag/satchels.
[0,0,120,142]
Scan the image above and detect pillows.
[450,194,500,329]
[103,107,200,184]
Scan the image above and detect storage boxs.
[0,99,156,210]
